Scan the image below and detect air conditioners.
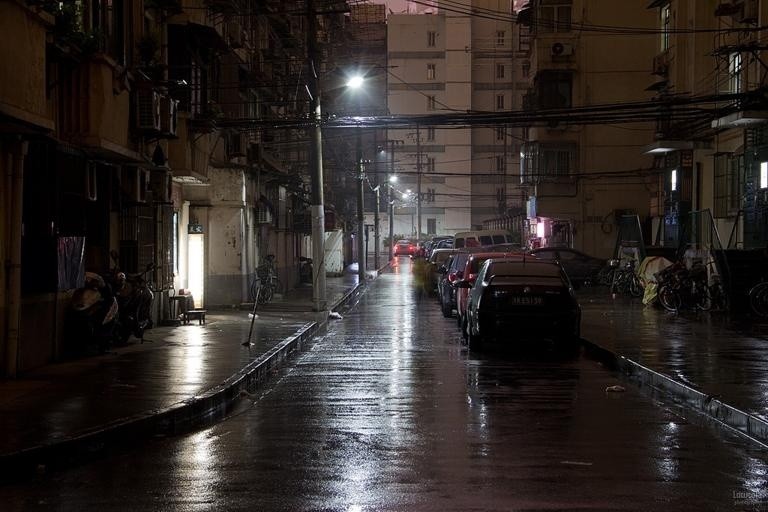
[552,41,572,56]
[123,163,149,206]
[161,95,177,140]
[137,89,161,132]
[256,205,273,223]
[151,167,175,206]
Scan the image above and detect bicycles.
[250,250,281,307]
[600,257,767,318]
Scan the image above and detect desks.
[169,295,191,325]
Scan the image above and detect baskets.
[653,269,673,286]
[689,264,706,280]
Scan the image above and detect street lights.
[308,74,368,312]
[386,193,409,262]
[374,175,398,268]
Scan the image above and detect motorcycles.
[67,263,131,360]
[108,250,161,350]
[297,255,312,285]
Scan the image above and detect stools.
[186,310,206,325]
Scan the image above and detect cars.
[415,222,585,360]
[523,245,605,291]
[392,237,414,257]
